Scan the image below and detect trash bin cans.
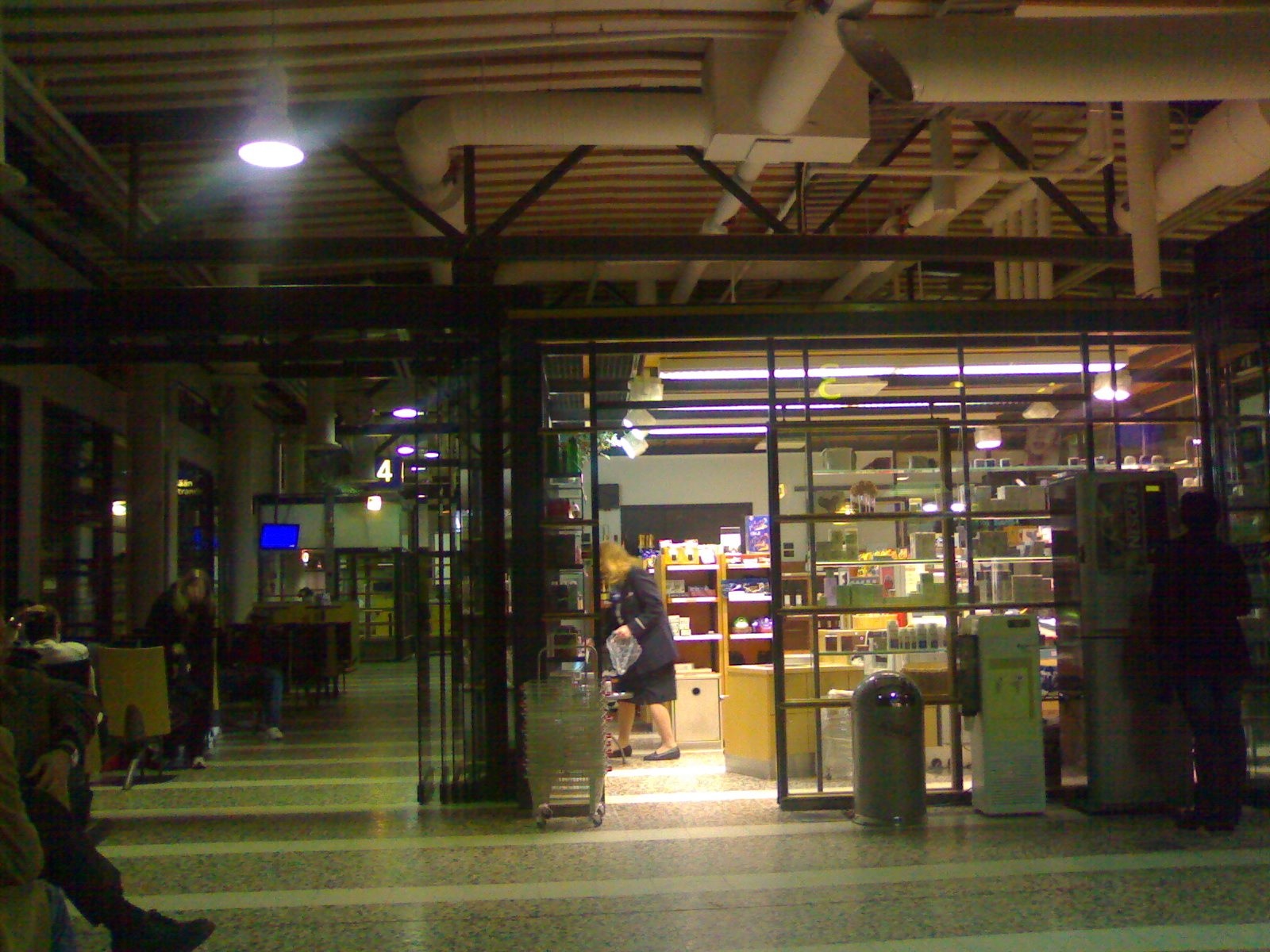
[850,670,928,827]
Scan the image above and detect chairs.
[92,644,178,790]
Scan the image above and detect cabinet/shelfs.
[654,456,1197,780]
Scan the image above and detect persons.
[141,569,223,769]
[229,603,285,742]
[0,605,217,952]
[599,540,681,761]
[1150,491,1254,834]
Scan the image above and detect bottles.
[887,621,950,649]
[825,570,837,608]
[784,580,802,606]
[828,528,860,561]
[615,531,656,573]
[558,436,579,474]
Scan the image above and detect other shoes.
[109,908,216,952]
[644,746,680,760]
[1170,804,1237,830]
[612,743,632,758]
[257,726,283,740]
[192,756,207,768]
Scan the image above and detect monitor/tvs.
[258,523,301,551]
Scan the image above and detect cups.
[825,448,851,469]
[1069,455,1166,466]
[973,458,1009,468]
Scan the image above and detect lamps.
[1092,373,1132,401]
[973,426,1002,449]
[621,370,663,460]
[235,0,306,169]
